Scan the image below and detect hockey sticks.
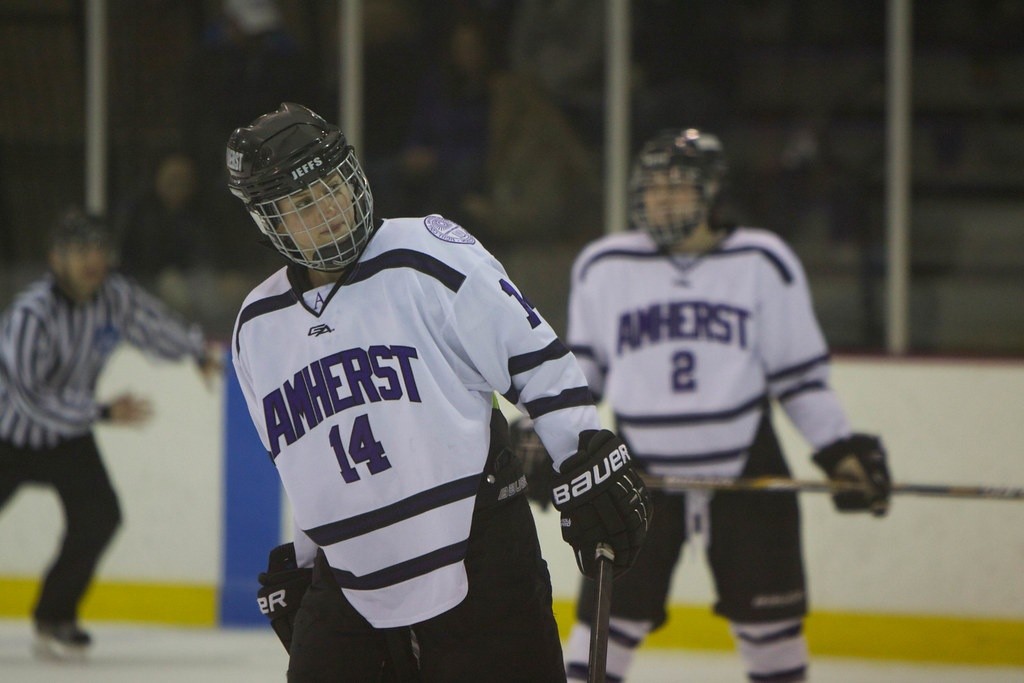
[584,538,616,683]
[642,470,1022,501]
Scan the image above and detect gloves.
[549,430,653,580]
[258,542,314,655]
[509,417,551,511]
[811,433,891,517]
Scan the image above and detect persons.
[227,103,651,683]
[125,155,217,325]
[400,17,494,237]
[509,132,891,683]
[0,208,222,655]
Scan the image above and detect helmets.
[627,129,731,247]
[50,211,113,301]
[225,102,376,272]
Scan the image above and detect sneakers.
[34,615,91,647]
